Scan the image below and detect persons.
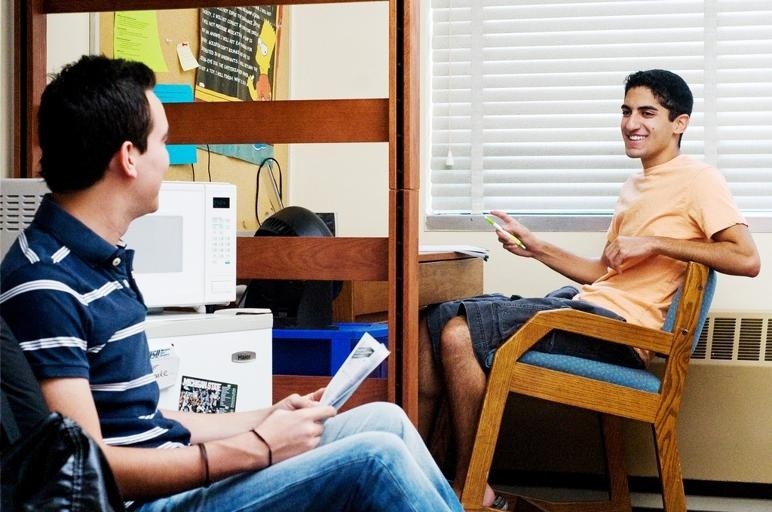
[0,54,466,512]
[418,69,761,511]
[179,387,222,413]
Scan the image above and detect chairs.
[456,235,716,511]
[0,318,50,447]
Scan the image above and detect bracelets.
[252,425,274,467]
[200,444,212,489]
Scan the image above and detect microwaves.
[0,177,249,314]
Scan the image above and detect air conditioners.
[613,311,772,486]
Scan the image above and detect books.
[418,243,491,264]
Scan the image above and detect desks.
[331,249,484,441]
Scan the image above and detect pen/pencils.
[483,215,527,250]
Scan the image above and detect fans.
[248,204,341,331]
